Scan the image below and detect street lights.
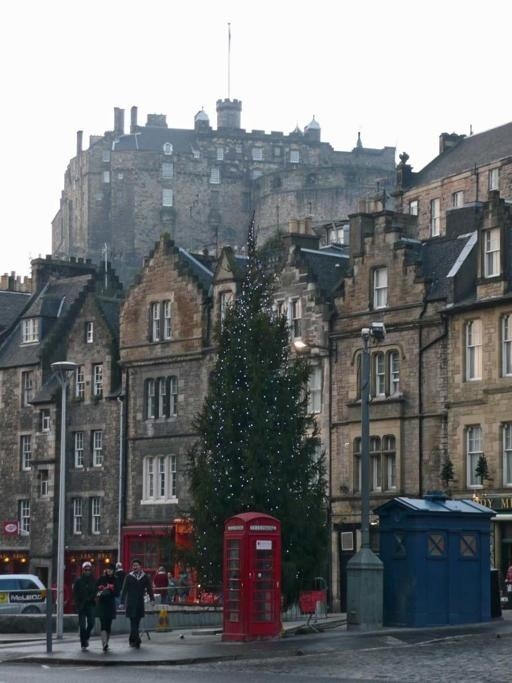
[346,322,386,632]
[50,359,77,641]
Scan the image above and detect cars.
[202,575,328,615]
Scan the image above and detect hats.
[82,562,92,568]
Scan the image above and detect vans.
[0,573,49,617]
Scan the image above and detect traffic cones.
[155,608,172,632]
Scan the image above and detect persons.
[119,559,155,649]
[115,562,126,589]
[154,566,189,604]
[73,561,98,651]
[96,564,121,651]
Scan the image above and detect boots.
[100,629,108,649]
